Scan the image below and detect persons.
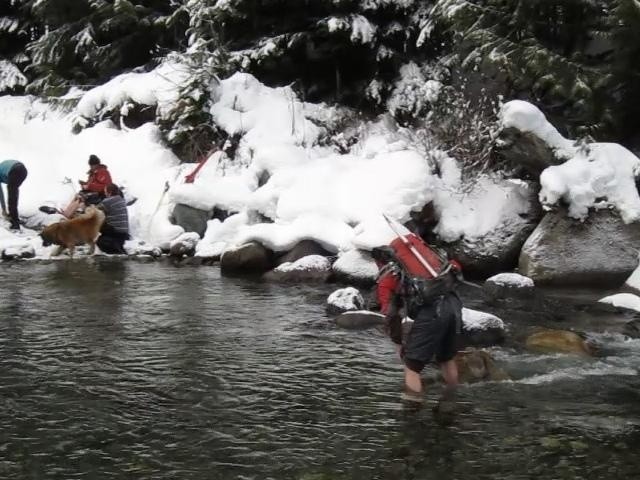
[96,184,129,237]
[370,215,465,393]
[74,155,112,213]
[0,159,27,229]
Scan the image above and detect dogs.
[37,204,107,258]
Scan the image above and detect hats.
[89,155,100,165]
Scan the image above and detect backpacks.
[375,234,464,320]
